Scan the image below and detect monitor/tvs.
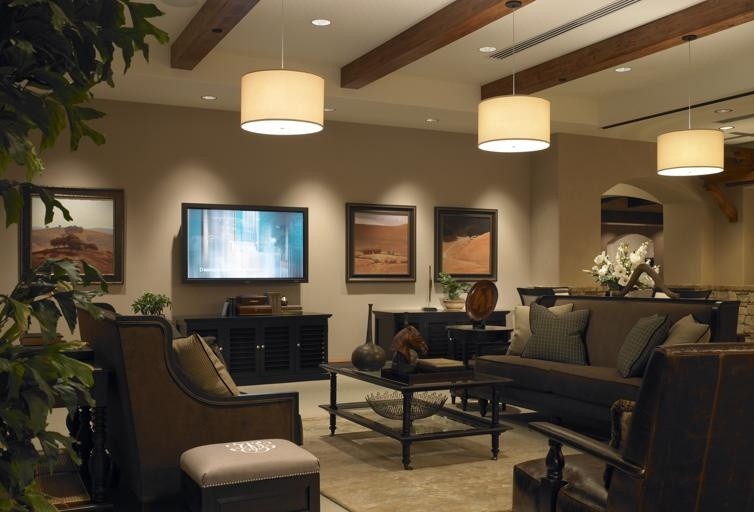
[181,203,309,285]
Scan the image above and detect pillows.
[520,301,590,366]
[602,398,636,493]
[617,311,671,378]
[663,312,711,345]
[171,331,240,398]
[506,304,573,357]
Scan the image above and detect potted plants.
[437,272,473,312]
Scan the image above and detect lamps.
[478,0,550,154]
[240,1,324,136]
[657,34,724,177]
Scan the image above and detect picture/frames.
[20,187,125,285]
[344,201,417,283]
[434,207,498,282]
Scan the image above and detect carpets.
[301,426,517,512]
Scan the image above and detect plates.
[465,279,499,321]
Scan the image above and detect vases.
[610,289,628,297]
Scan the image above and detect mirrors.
[600,182,666,284]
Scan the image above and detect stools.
[180,438,320,512]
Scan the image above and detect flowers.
[581,240,661,291]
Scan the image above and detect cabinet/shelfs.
[446,325,511,416]
[374,308,513,359]
[173,311,333,386]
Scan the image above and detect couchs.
[477,299,741,441]
[513,342,754,512]
[74,302,304,511]
